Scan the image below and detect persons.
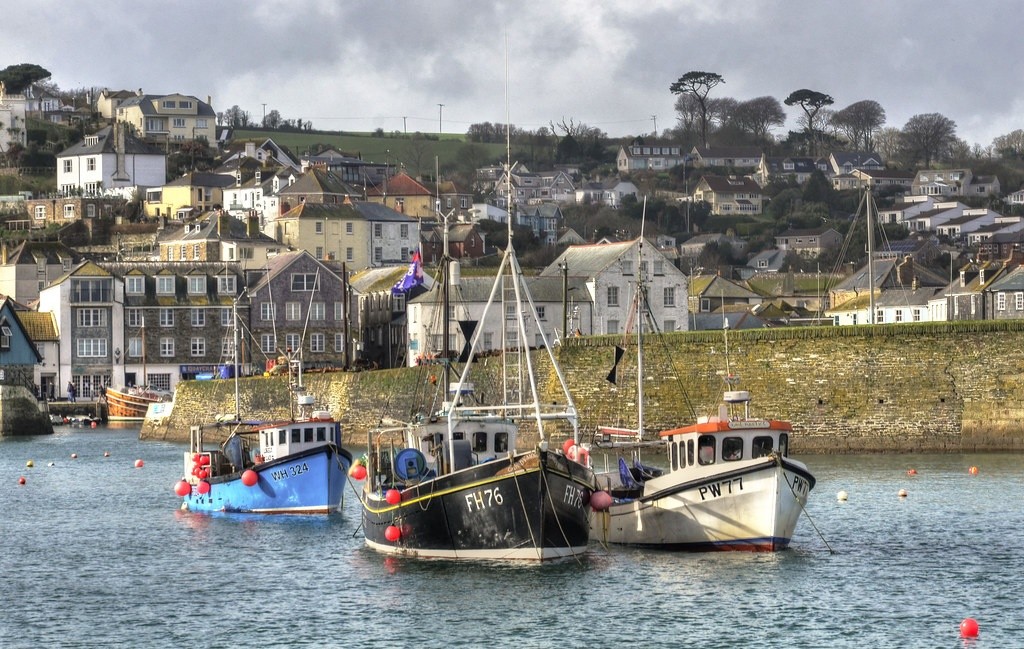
[49,381,56,402]
[98,385,107,403]
[66,381,78,402]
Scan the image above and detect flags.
[391,242,430,301]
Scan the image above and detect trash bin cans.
[217,365,241,379]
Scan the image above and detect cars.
[127,381,174,398]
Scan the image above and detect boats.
[46,412,102,427]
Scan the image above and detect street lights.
[260,103,267,129]
[437,103,446,133]
[401,116,408,135]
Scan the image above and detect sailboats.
[587,190,817,555]
[360,118,595,571]
[100,317,172,422]
[181,254,354,516]
